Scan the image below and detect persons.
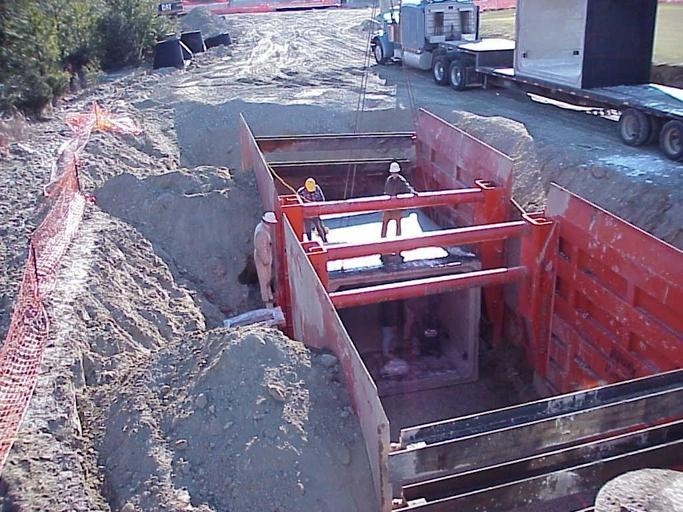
[297,178,329,246]
[254,212,278,303]
[381,162,419,238]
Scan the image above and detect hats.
[304,178,315,192]
[261,211,279,224]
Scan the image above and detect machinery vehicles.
[153,0,185,18]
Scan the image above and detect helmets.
[388,163,400,173]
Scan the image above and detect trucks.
[370,0,683,164]
